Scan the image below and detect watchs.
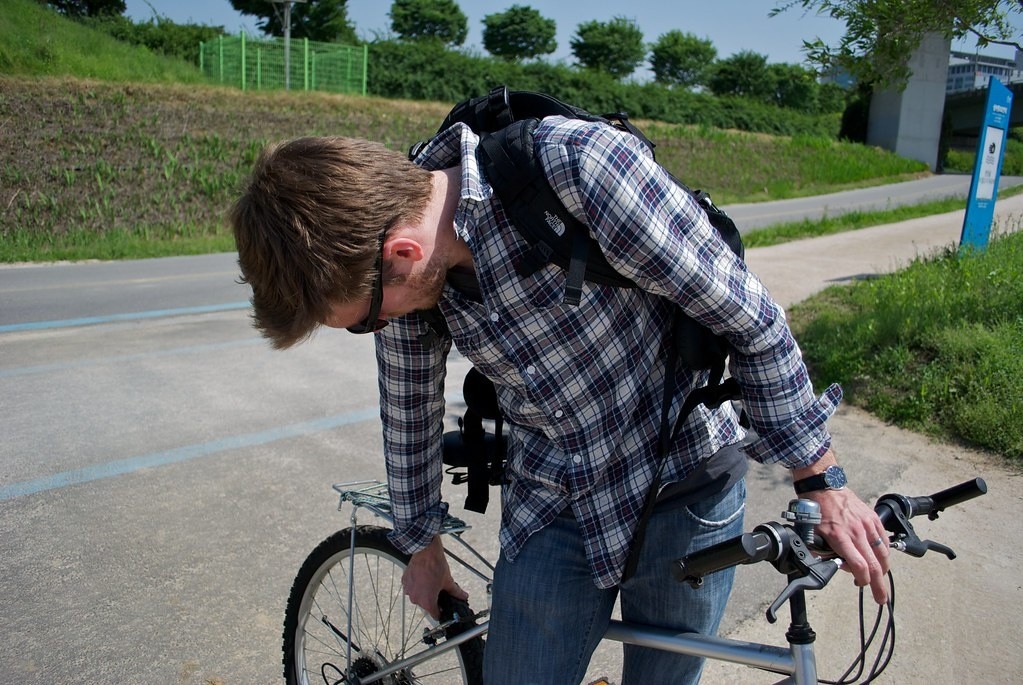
[791,465,849,494]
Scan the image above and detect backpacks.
[407,84,745,421]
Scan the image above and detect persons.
[227,109,895,685]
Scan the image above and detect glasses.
[345,214,390,335]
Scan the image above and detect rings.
[871,537,884,548]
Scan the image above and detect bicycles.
[282,476,987,684]
